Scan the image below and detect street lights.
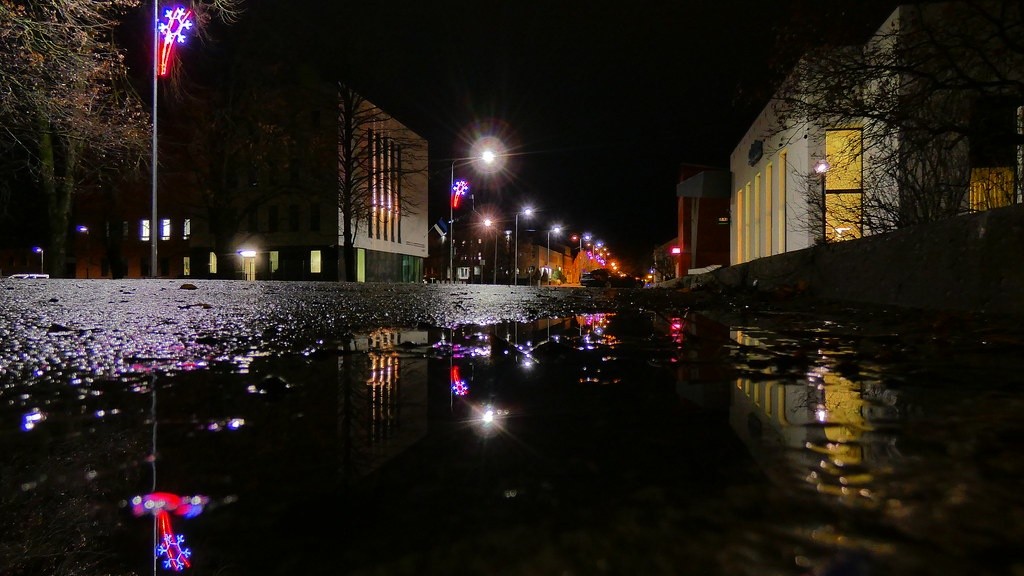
[515,206,534,286]
[450,136,507,280]
[592,240,605,279]
[580,232,592,285]
[547,223,562,283]
[478,214,497,284]
